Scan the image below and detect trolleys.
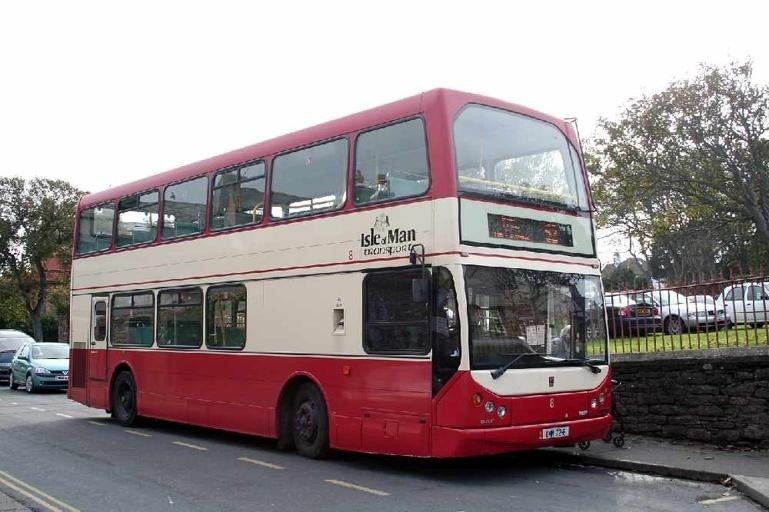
[576,379,627,451]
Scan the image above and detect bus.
[64,84,615,465]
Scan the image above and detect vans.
[0,328,44,383]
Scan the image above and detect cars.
[574,280,769,342]
[8,341,72,394]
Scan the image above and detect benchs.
[85,206,271,253]
[344,169,429,207]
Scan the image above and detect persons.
[466,304,487,335]
[535,324,579,359]
[335,170,370,210]
